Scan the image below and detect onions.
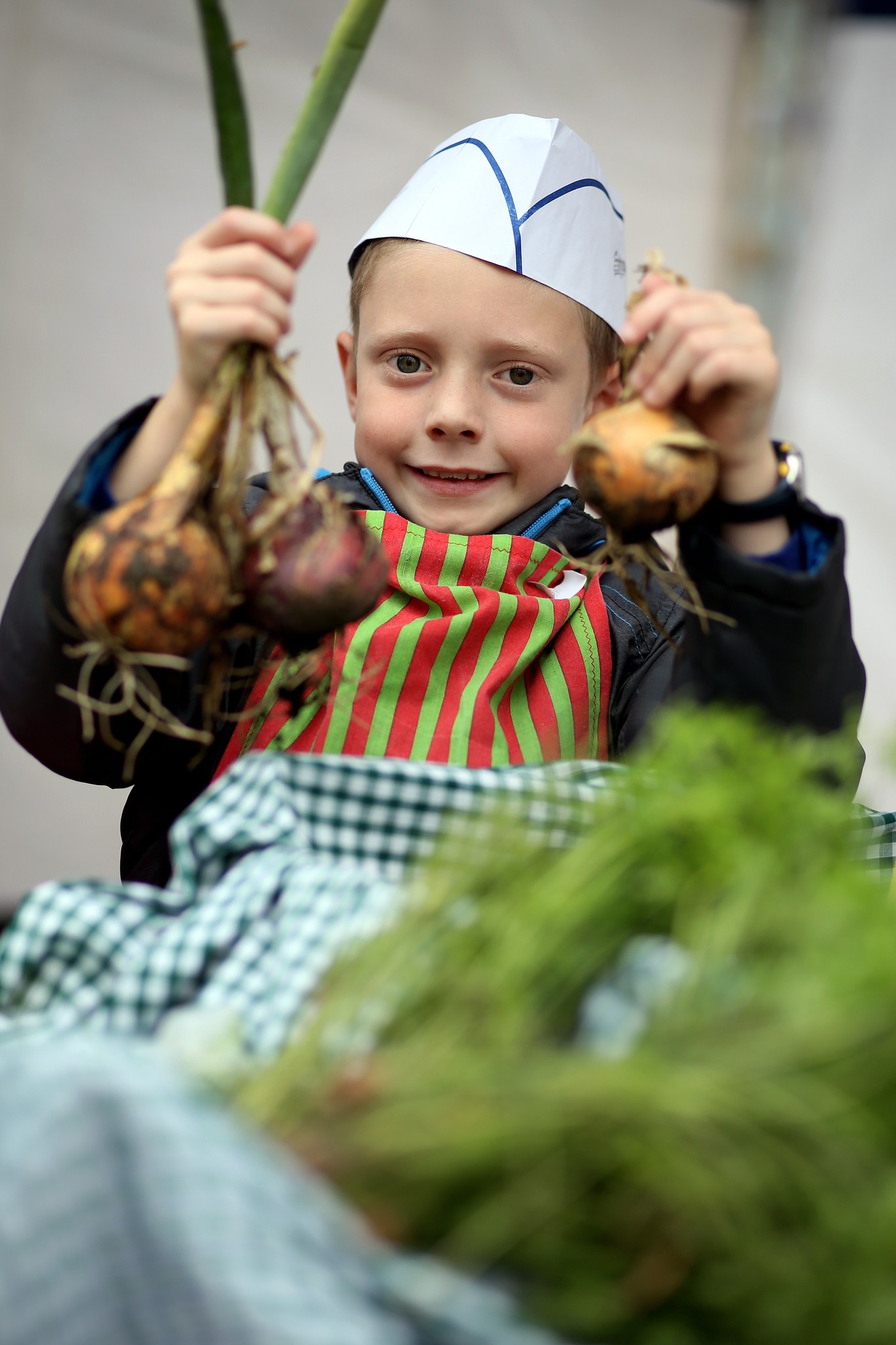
[61,5,320,778]
[172,28,391,740]
[532,380,724,557]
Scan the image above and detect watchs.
[695,437,811,527]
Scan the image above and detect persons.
[4,110,869,1002]
